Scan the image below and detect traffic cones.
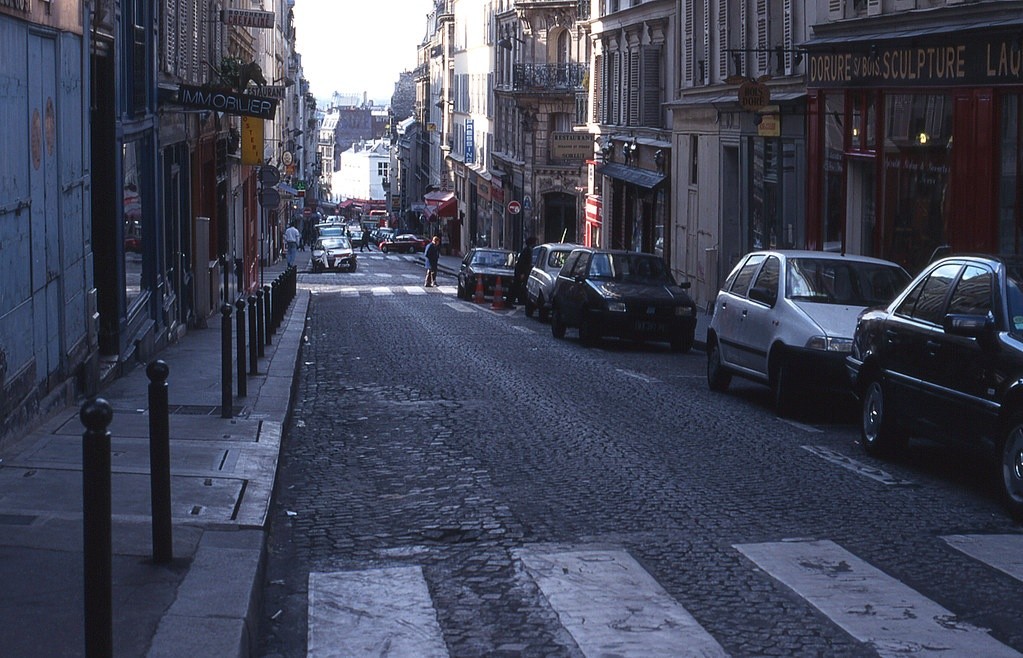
[490,276,506,310]
[473,275,486,303]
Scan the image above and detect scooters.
[313,245,358,273]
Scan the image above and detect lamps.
[498,36,525,51]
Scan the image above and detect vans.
[705,250,916,414]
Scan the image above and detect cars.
[520,243,612,322]
[458,248,519,302]
[552,248,698,354]
[364,210,418,245]
[379,234,432,254]
[311,237,354,269]
[315,215,364,246]
[843,244,1023,525]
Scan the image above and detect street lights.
[269,77,297,267]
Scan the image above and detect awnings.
[434,196,457,216]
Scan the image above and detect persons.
[424,236,441,288]
[284,223,299,270]
[360,229,373,252]
